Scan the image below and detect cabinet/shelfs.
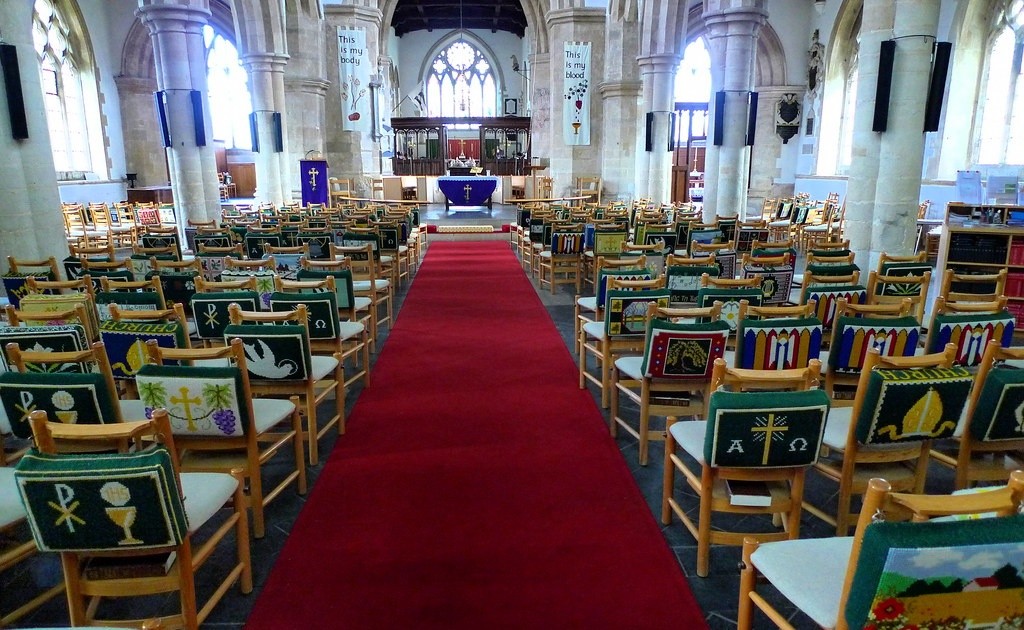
[215,140,257,198]
[931,202,1024,340]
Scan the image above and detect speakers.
[0,45,29,140]
[747,92,759,146]
[273,113,283,152]
[249,112,258,152]
[922,42,953,132]
[872,41,896,132]
[645,113,653,151]
[190,90,206,146]
[669,113,676,150]
[714,92,725,145]
[153,91,170,147]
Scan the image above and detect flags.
[563,41,591,146]
[336,26,371,132]
[408,81,426,111]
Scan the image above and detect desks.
[125,187,174,205]
[436,176,498,211]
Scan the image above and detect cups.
[486,170,490,176]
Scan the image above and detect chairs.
[510,175,1024,630]
[0,173,428,630]
